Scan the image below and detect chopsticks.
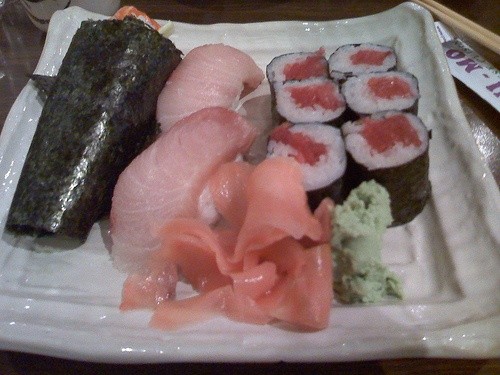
[412,0,499,56]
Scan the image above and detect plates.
[1,6,499,364]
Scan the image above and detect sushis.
[110,43,431,277]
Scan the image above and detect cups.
[20,0,120,31]
